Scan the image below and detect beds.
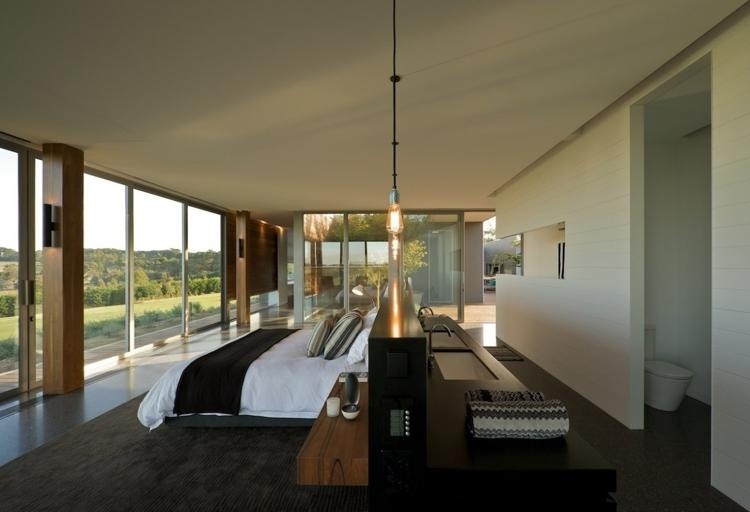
[162,312,379,429]
[338,277,384,305]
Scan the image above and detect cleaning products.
[516,260,522,275]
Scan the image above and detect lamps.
[385,0,406,236]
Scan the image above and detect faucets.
[418,305,435,317]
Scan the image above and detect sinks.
[433,349,500,384]
[426,329,469,349]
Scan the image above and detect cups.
[326,397,341,418]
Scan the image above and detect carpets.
[483,345,524,361]
[0,390,367,511]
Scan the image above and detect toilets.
[643,323,694,412]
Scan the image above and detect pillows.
[305,310,378,365]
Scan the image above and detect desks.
[295,373,368,487]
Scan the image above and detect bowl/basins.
[341,404,361,420]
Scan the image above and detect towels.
[465,389,570,441]
